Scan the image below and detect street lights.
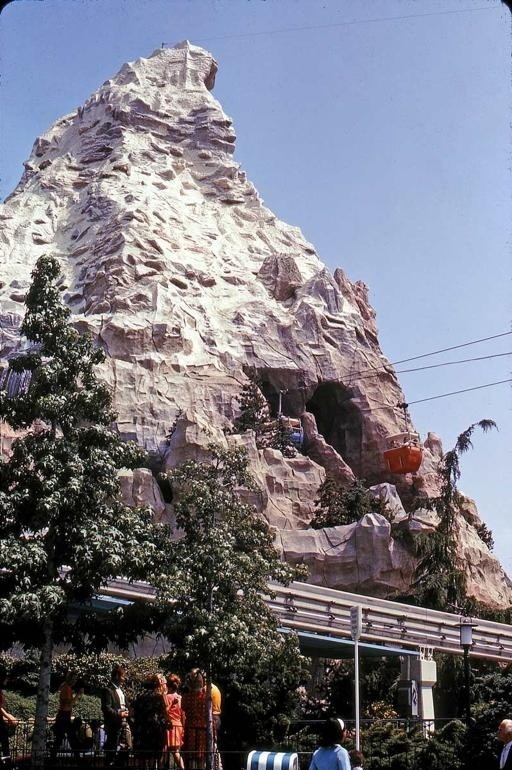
[453,617,476,769]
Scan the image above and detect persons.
[45,664,225,770]
[306,715,352,770]
[386,437,419,447]
[347,749,366,770]
[494,718,512,770]
[1,689,19,770]
